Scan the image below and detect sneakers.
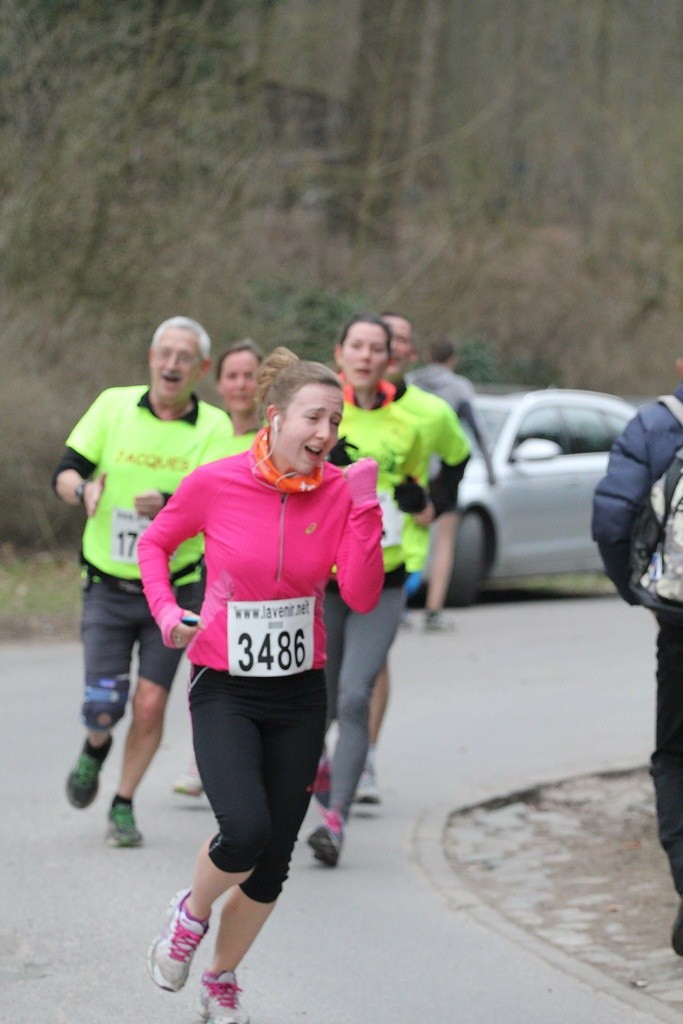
[66,734,112,808]
[105,803,142,846]
[312,764,331,808]
[308,801,346,866]
[197,969,249,1024]
[147,887,212,991]
[354,756,380,803]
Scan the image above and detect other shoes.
[672,901,683,956]
[174,762,204,794]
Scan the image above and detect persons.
[171,338,266,795]
[352,313,475,805]
[307,316,430,868]
[50,318,234,847]
[408,334,497,632]
[592,350,683,958]
[137,346,384,1024]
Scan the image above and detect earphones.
[274,415,280,433]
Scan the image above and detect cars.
[406,388,642,609]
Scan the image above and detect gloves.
[392,475,427,511]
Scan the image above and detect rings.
[177,636,181,642]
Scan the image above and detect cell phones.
[181,617,198,627]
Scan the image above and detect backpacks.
[628,394,683,628]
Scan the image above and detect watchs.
[76,479,89,502]
